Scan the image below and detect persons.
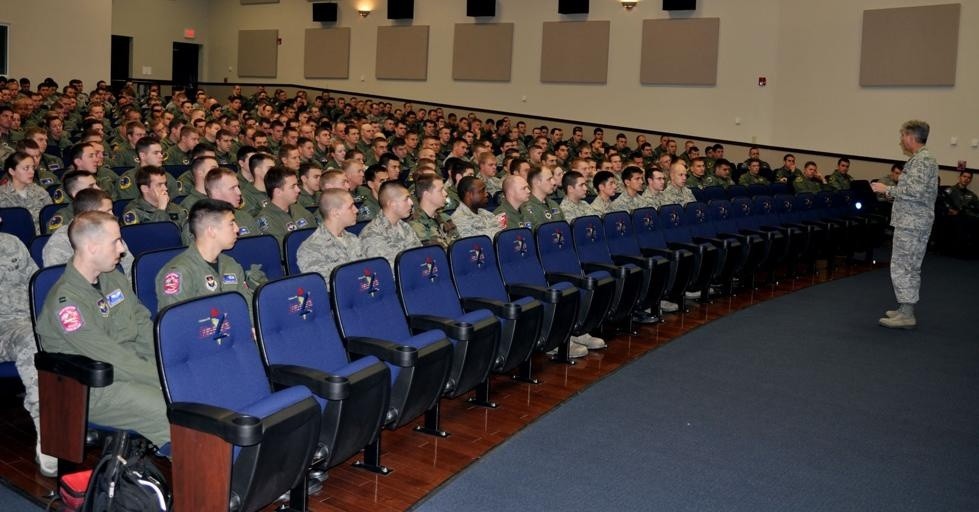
[876,161,906,203]
[869,119,939,330]
[0,76,855,483]
[943,170,979,215]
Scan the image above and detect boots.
[879,304,916,329]
[32,416,59,478]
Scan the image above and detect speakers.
[663,0,696,10]
[313,3,337,22]
[467,0,496,16]
[387,0,414,19]
[558,0,589,14]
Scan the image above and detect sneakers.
[661,299,679,312]
[570,334,606,349]
[546,339,589,357]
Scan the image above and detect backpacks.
[82,429,172,512]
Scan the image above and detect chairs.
[4,359,19,383]
[131,246,192,321]
[445,235,543,409]
[329,256,457,477]
[395,245,504,436]
[599,210,669,323]
[702,179,893,306]
[570,215,642,349]
[631,206,692,313]
[3,165,699,274]
[492,227,578,386]
[152,290,321,509]
[28,259,167,509]
[252,272,390,510]
[220,233,283,297]
[534,219,614,365]
